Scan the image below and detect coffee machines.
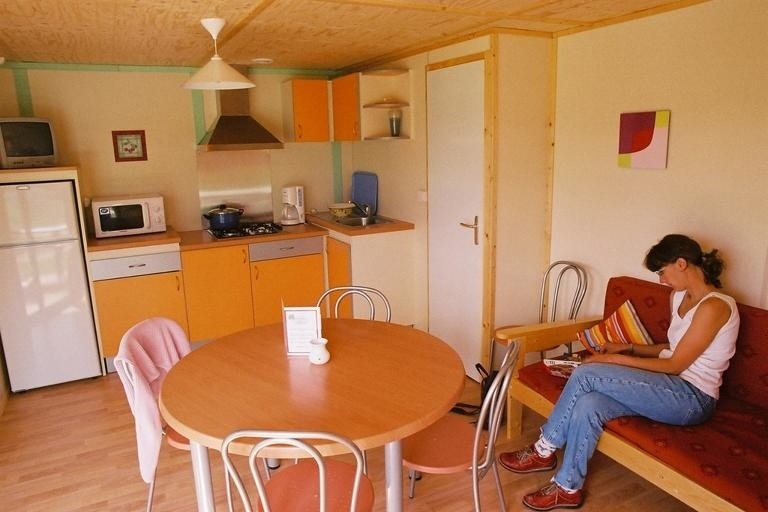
[279,185,305,226]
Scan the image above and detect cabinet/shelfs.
[332,68,414,141]
[281,79,330,143]
[87,238,190,377]
[327,223,353,319]
[180,230,326,343]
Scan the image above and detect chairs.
[294,286,392,478]
[221,430,374,512]
[488,261,588,377]
[114,316,235,512]
[401,341,521,510]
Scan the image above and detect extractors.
[196,63,285,153]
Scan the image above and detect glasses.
[656,267,666,276]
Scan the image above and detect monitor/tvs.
[0,117,58,169]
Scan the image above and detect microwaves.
[91,193,167,240]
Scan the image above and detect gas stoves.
[206,221,283,242]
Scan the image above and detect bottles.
[307,337,331,366]
[388,110,402,137]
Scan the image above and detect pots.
[202,204,244,231]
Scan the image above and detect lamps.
[181,17,256,91]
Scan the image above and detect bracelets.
[631,343,633,353]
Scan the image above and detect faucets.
[349,200,372,216]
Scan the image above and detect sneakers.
[522,482,582,511]
[498,444,557,473]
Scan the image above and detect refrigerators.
[0,165,107,394]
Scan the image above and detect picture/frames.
[112,130,148,162]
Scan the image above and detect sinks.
[333,215,393,229]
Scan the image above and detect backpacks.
[450,364,505,430]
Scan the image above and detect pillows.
[577,298,655,356]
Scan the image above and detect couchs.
[497,276,768,511]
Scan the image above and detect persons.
[497,235,740,509]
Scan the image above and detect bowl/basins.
[327,203,357,218]
